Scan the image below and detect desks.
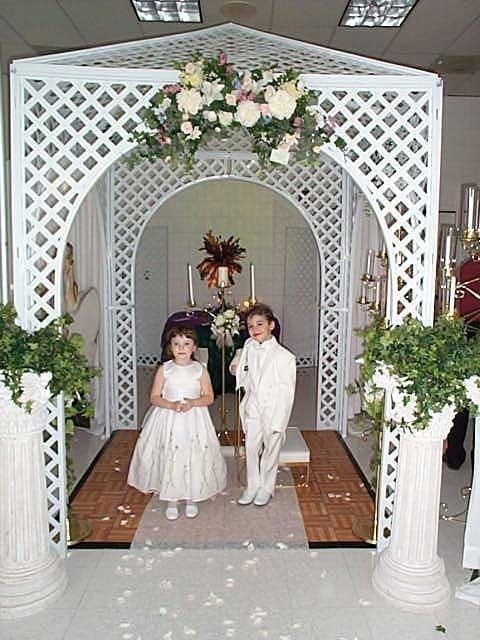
[164,313,280,397]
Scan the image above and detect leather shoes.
[254,488,273,506]
[237,487,258,505]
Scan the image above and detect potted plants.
[344,312,480,604]
[0,301,108,618]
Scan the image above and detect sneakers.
[166,507,178,520]
[184,503,199,518]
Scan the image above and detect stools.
[274,427,312,488]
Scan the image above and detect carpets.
[63,429,381,549]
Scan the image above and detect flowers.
[196,228,249,291]
[129,47,330,175]
[204,308,247,351]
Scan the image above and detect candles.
[360,240,385,306]
[445,235,451,269]
[251,264,255,304]
[188,264,195,305]
[217,266,230,288]
[467,187,475,233]
[449,275,456,314]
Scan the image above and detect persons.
[228,304,297,506]
[62,241,79,323]
[126,324,229,520]
